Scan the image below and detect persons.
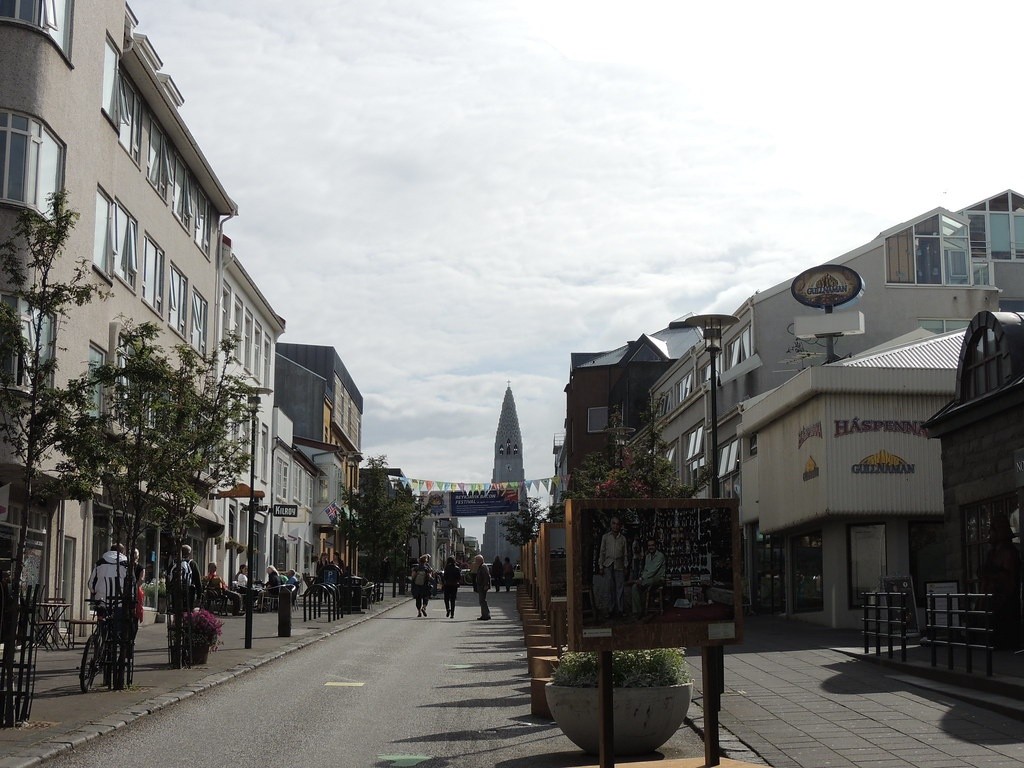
[491,556,504,592]
[206,550,351,616]
[441,555,462,619]
[411,555,434,617]
[472,554,492,620]
[165,544,202,628]
[597,517,667,621]
[502,557,515,592]
[87,543,145,669]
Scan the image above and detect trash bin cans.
[398,572,408,594]
[340,575,363,612]
[278,587,292,637]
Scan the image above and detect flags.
[325,501,343,521]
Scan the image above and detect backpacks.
[169,560,194,587]
[414,566,426,585]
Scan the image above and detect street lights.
[685,314,738,695]
[604,427,635,470]
[237,386,273,650]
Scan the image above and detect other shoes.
[233,611,245,616]
[421,608,427,617]
[632,607,645,621]
[450,617,454,619]
[418,614,421,617]
[652,510,712,575]
[254,608,261,611]
[477,616,491,620]
[447,609,450,617]
[605,613,614,618]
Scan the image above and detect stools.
[582,585,596,624]
[645,585,664,615]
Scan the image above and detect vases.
[188,646,210,664]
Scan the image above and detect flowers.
[166,607,226,654]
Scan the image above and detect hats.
[103,551,127,564]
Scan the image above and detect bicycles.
[75,598,128,694]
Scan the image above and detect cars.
[438,568,477,586]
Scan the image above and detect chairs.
[202,578,229,617]
[231,581,302,612]
[18,598,99,652]
[362,581,375,610]
[300,572,319,597]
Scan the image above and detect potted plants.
[544,647,695,758]
[142,578,167,612]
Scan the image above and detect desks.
[33,604,72,651]
